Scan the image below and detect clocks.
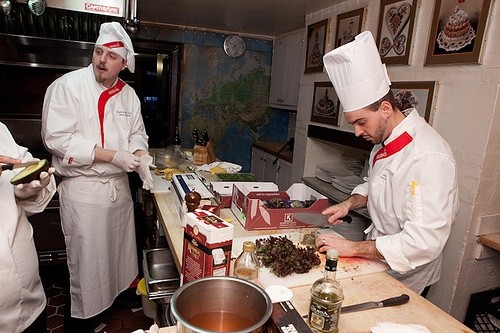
[223,34,246,59]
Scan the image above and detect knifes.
[291,214,353,229]
[191,128,209,147]
[0,161,40,171]
[302,293,410,318]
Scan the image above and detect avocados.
[10,159,49,185]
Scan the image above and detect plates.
[197,159,258,182]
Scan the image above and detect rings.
[323,239,325,244]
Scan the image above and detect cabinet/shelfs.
[251,147,292,191]
[270,30,303,107]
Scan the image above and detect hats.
[323,31,392,112]
[95,22,135,73]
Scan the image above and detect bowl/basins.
[154,151,179,169]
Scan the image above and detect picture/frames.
[310,81,340,127]
[376,0,421,66]
[334,5,368,49]
[423,0,495,67]
[391,81,440,126]
[303,16,332,74]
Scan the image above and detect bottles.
[232,240,259,286]
[308,247,344,333]
[173,127,181,153]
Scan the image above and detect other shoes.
[113,297,140,308]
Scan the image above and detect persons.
[314,31,459,299]
[0,121,56,333]
[42,21,157,333]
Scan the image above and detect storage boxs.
[230,183,329,231]
[170,173,214,228]
[180,210,234,284]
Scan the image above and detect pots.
[168,275,272,333]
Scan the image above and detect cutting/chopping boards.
[229,229,391,288]
[287,272,475,333]
[147,167,187,194]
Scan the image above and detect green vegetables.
[255,235,321,277]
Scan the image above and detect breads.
[166,170,185,180]
[210,167,226,175]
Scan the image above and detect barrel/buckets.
[137,277,156,318]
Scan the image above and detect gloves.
[136,155,157,190]
[110,150,141,172]
[14,167,55,199]
